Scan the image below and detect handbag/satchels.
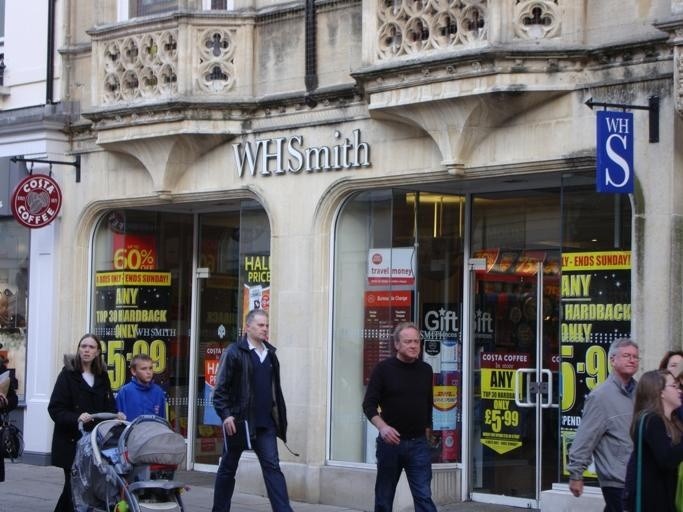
[0,413,23,458]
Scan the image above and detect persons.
[657,348,681,390]
[0,355,21,490]
[113,351,166,426]
[44,330,117,512]
[567,336,638,512]
[361,321,440,512]
[625,368,683,512]
[210,309,294,512]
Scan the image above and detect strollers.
[71,411,187,512]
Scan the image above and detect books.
[221,419,253,453]
